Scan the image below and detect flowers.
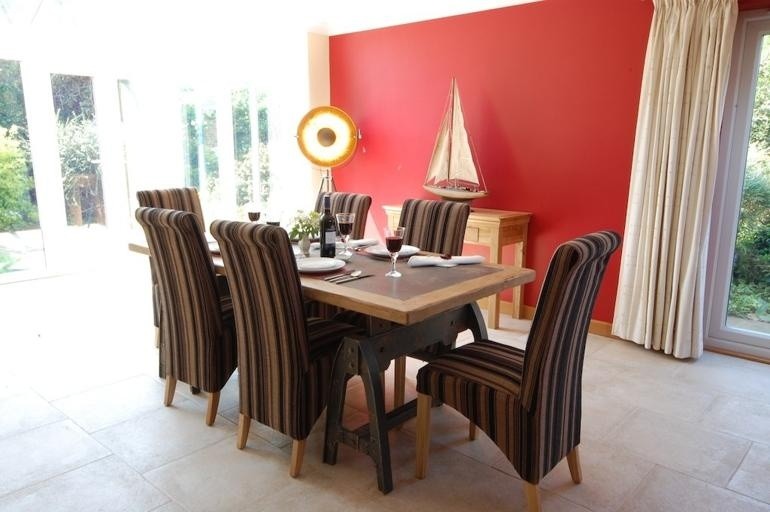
[290,207,321,239]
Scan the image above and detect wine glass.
[382,226,404,278]
[336,212,356,258]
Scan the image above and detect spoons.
[329,270,363,283]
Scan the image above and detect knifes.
[334,268,374,285]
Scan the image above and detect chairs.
[137,186,210,235]
[412,224,621,511]
[395,195,470,257]
[136,203,240,427]
[312,192,374,246]
[212,215,364,481]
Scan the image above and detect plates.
[204,231,220,254]
[364,244,419,257]
[294,257,347,272]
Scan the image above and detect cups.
[247,208,261,223]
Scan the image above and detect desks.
[382,204,532,331]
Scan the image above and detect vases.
[299,233,314,253]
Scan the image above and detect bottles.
[319,195,335,258]
